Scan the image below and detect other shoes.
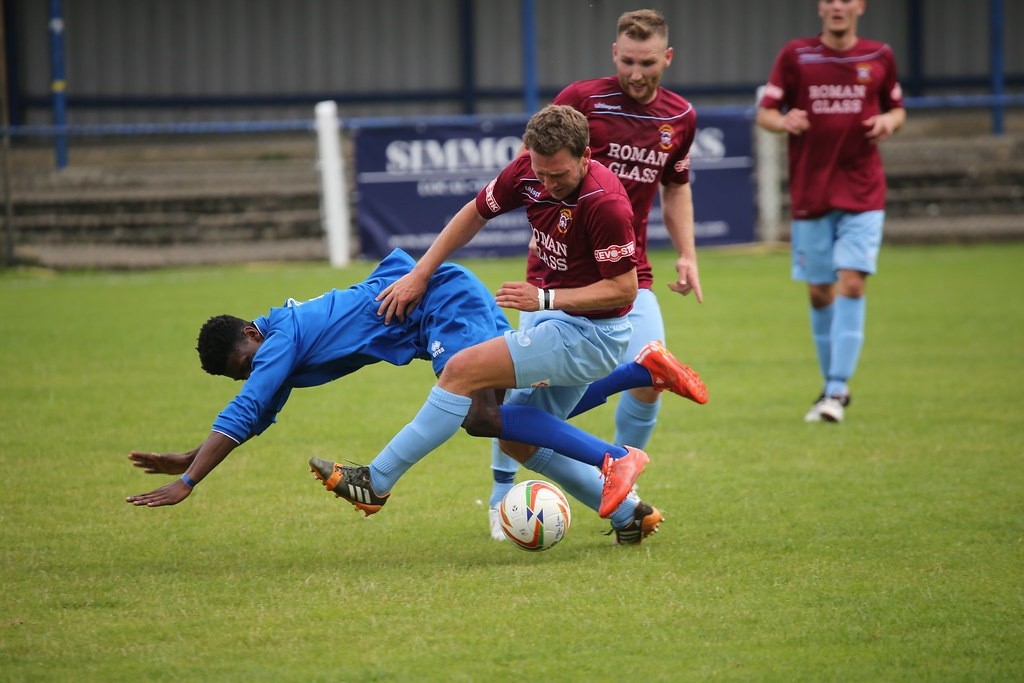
[488,498,506,541]
[820,392,849,422]
[804,391,824,425]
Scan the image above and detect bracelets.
[181,473,195,488]
[538,289,555,310]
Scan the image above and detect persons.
[308,106,665,545]
[489,9,702,540]
[126,247,709,506]
[756,0,907,423]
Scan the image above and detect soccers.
[498,478,572,553]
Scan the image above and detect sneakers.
[634,338,708,404]
[599,444,650,519]
[600,500,665,546]
[309,457,391,518]
